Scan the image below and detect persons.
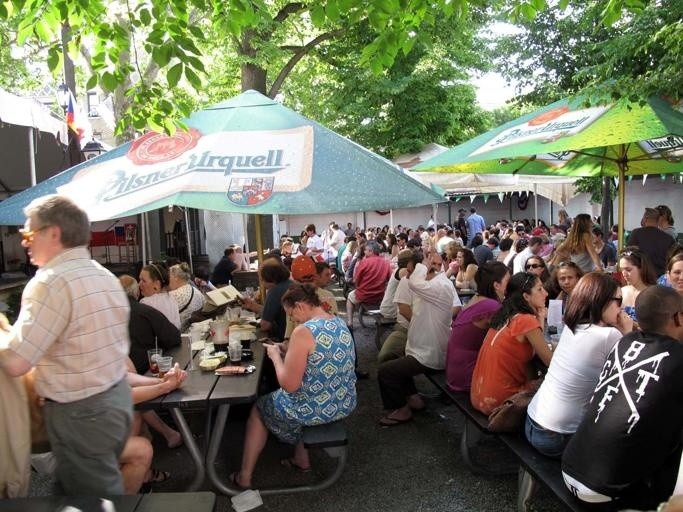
[554,262,584,305]
[0,194,135,512]
[394,240,407,249]
[582,205,676,228]
[128,356,184,448]
[471,273,556,418]
[455,249,481,293]
[647,256,665,278]
[345,240,394,331]
[455,207,515,228]
[118,274,183,375]
[515,209,582,227]
[584,262,614,272]
[560,285,682,512]
[665,255,682,294]
[376,240,394,258]
[340,241,367,282]
[234,258,299,343]
[376,249,455,428]
[284,256,340,287]
[377,250,463,365]
[455,228,683,254]
[168,262,207,335]
[380,252,413,319]
[522,257,555,298]
[140,262,183,334]
[284,256,340,353]
[23,363,189,496]
[473,255,535,273]
[407,214,455,250]
[614,246,660,333]
[555,257,615,271]
[524,272,639,460]
[279,221,340,257]
[446,261,513,393]
[214,243,259,285]
[355,368,370,380]
[229,282,359,490]
[441,242,464,281]
[340,222,407,240]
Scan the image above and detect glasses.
[19,225,52,241]
[289,312,295,323]
[620,251,641,262]
[522,272,533,291]
[612,296,622,307]
[560,262,576,269]
[526,264,542,268]
[657,206,666,210]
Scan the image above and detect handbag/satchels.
[488,379,544,432]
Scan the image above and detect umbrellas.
[0,90,453,306]
[407,86,683,256]
[515,175,582,228]
[376,141,520,230]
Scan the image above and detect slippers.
[410,400,426,410]
[377,409,411,427]
[280,458,312,472]
[227,472,251,490]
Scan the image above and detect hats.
[458,208,467,213]
[398,249,413,260]
[292,256,317,282]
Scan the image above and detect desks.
[0,490,216,512]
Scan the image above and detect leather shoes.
[355,369,369,378]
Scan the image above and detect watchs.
[192,269,217,297]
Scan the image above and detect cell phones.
[258,337,275,346]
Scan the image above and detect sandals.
[167,435,197,449]
[143,469,170,484]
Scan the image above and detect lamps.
[85,87,102,122]
[80,137,107,164]
[56,79,70,119]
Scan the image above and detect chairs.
[117,227,137,264]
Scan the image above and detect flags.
[66,90,87,147]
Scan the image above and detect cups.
[547,299,562,326]
[227,344,242,362]
[240,332,251,347]
[549,333,560,351]
[155,356,173,378]
[146,348,162,373]
[209,320,229,351]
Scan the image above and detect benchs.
[357,299,397,351]
[331,266,357,299]
[269,420,349,493]
[423,369,626,512]
